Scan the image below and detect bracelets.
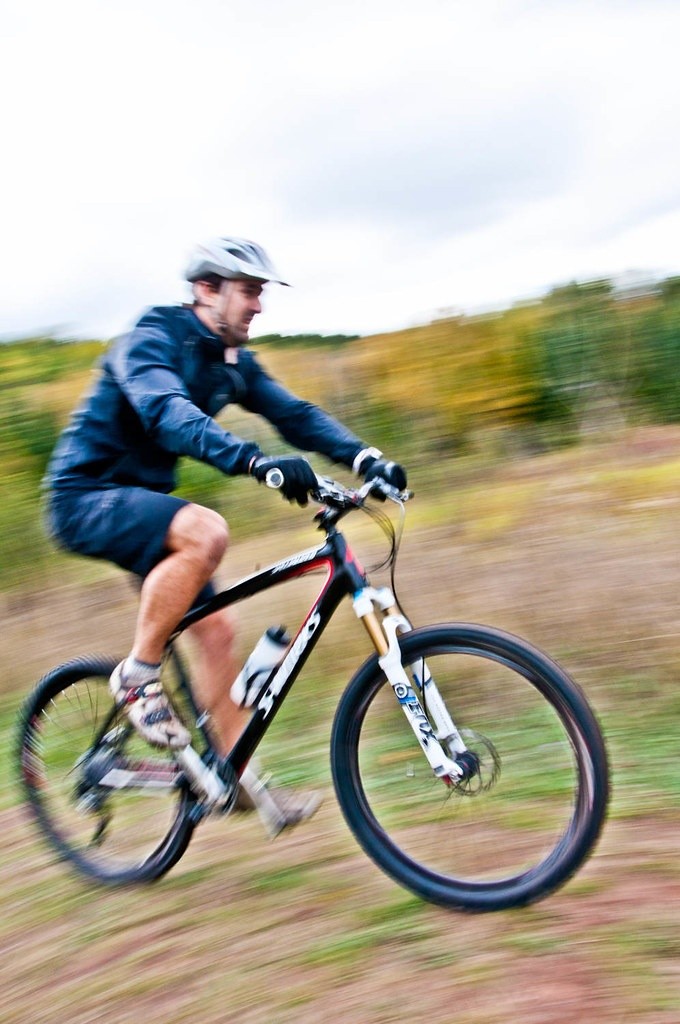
[247,452,262,475]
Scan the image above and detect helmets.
[184,236,292,288]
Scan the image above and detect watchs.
[354,447,384,475]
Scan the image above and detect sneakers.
[110,656,191,748]
[239,787,321,823]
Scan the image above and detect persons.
[40,244,411,838]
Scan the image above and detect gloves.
[364,455,408,502]
[254,454,316,508]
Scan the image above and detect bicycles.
[15,462,614,913]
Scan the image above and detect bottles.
[228,625,290,706]
